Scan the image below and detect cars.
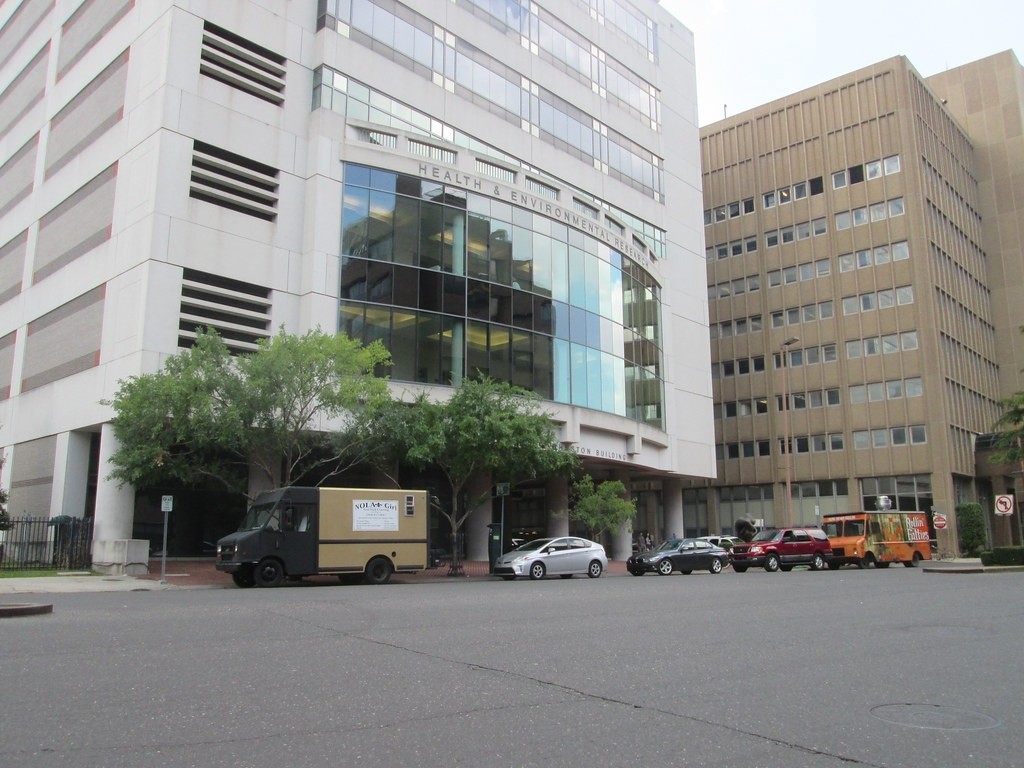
[696,534,745,568]
[493,536,609,580]
[626,538,729,576]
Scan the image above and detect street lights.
[780,336,805,529]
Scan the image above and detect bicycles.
[932,544,956,561]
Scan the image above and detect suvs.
[728,526,834,573]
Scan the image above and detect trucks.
[821,510,932,569]
[215,486,429,587]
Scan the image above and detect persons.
[671,532,676,540]
[638,532,652,553]
